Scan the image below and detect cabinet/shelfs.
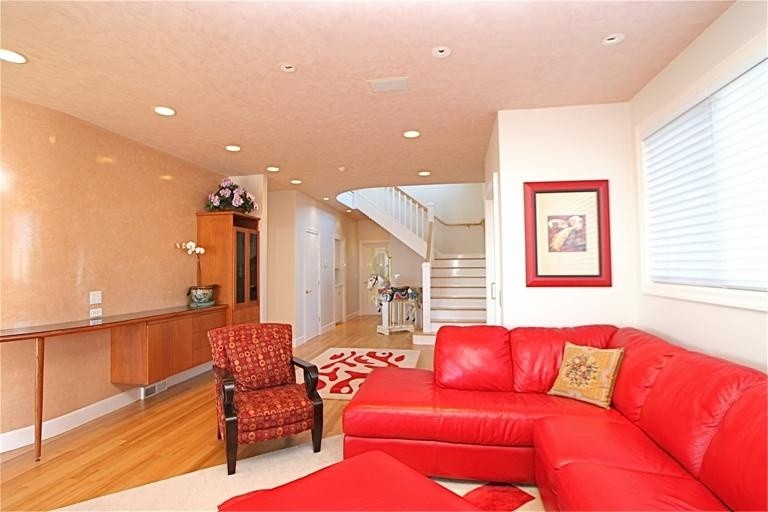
[196,211,261,328]
[0,302,228,461]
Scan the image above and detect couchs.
[340,323,613,483]
[534,327,756,511]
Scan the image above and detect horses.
[366,273,419,321]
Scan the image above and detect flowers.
[177,240,205,287]
[205,178,259,212]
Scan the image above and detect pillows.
[544,342,625,410]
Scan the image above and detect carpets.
[48,435,547,512]
[289,348,423,400]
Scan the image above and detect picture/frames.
[523,179,615,289]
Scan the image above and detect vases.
[189,284,219,308]
[219,200,245,213]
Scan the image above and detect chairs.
[206,322,323,473]
